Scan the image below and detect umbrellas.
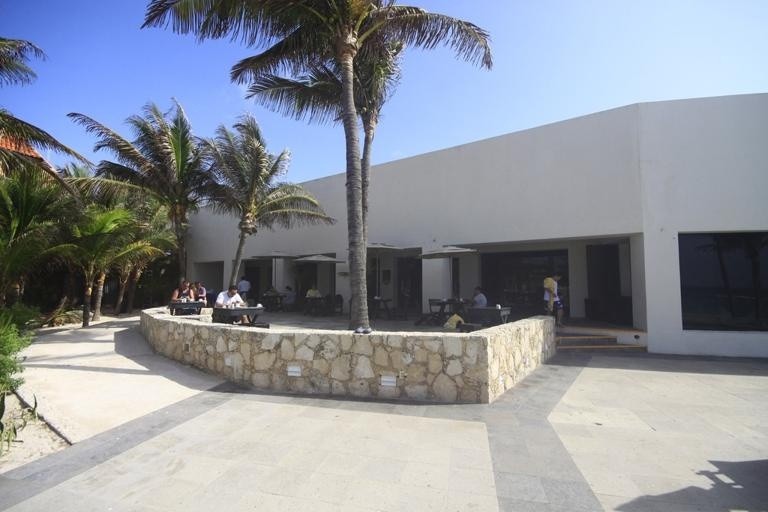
[419,247,481,317]
[293,255,346,288]
[250,250,298,290]
[345,242,407,297]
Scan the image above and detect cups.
[226,304,230,309]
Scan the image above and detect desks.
[169,291,287,328]
[413,296,512,332]
[302,291,393,319]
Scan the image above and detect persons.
[542,272,565,328]
[170,280,322,323]
[444,311,464,329]
[471,286,487,305]
